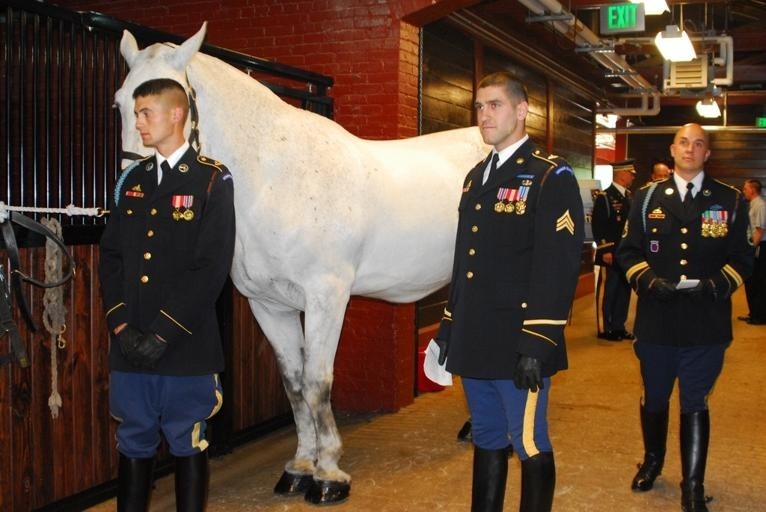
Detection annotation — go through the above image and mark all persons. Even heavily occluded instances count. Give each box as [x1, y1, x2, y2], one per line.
[96, 75, 234, 511]
[591, 156, 765, 342]
[612, 120, 759, 512]
[434, 64, 585, 511]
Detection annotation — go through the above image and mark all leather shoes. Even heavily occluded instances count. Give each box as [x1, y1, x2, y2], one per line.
[618, 329, 634, 340]
[746, 319, 765, 324]
[737, 315, 749, 320]
[598, 330, 622, 341]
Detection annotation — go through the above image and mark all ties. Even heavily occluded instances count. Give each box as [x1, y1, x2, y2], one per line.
[625, 189, 630, 200]
[159, 160, 172, 185]
[684, 182, 695, 206]
[488, 152, 499, 179]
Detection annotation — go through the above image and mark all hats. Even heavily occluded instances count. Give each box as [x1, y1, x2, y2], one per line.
[608, 159, 638, 175]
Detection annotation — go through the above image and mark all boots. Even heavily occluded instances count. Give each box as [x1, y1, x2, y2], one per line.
[116, 452, 153, 512]
[469, 446, 508, 512]
[520, 452, 555, 512]
[679, 409, 711, 512]
[631, 396, 669, 492]
[172, 448, 208, 512]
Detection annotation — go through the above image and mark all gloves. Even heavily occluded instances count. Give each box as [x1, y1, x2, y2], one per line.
[513, 356, 544, 393]
[433, 338, 449, 366]
[685, 276, 717, 306]
[115, 324, 158, 372]
[123, 331, 169, 368]
[649, 279, 680, 302]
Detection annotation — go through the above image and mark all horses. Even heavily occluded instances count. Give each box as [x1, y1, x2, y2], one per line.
[111, 21, 493, 506]
